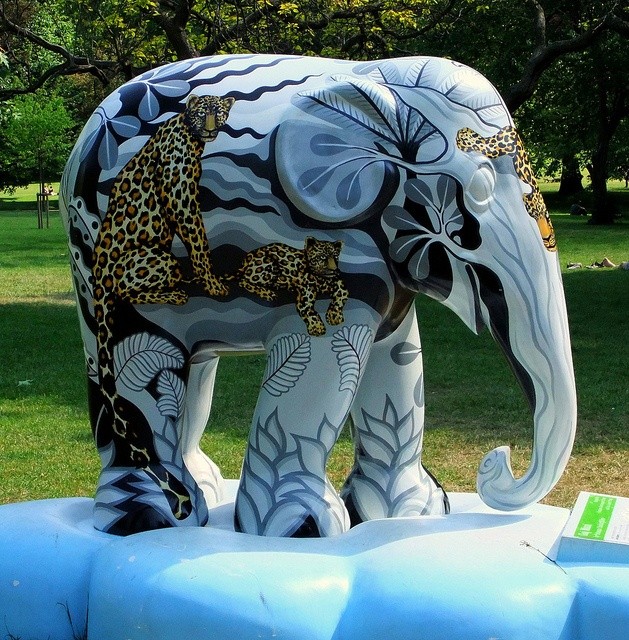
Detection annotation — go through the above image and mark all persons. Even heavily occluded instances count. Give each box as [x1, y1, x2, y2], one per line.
[601, 257, 629, 270]
